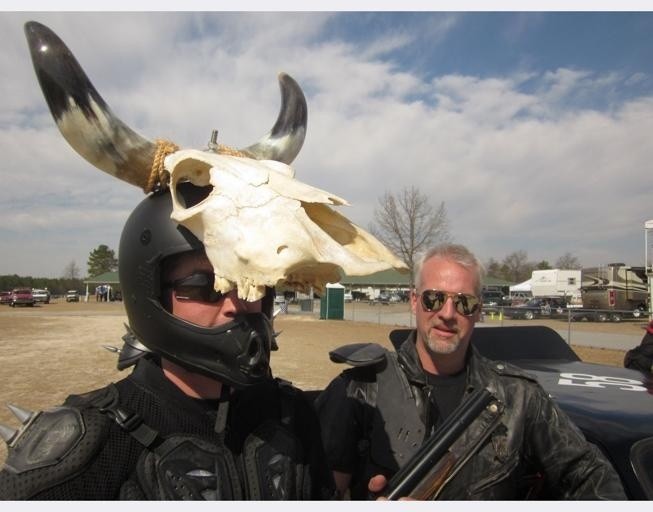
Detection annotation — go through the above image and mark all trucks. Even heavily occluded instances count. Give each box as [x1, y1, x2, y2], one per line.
[483, 263, 652, 323]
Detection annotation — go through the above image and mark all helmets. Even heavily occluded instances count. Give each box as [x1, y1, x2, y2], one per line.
[103, 185, 279, 390]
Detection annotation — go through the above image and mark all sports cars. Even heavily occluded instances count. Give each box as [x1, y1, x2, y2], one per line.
[358, 323, 652, 501]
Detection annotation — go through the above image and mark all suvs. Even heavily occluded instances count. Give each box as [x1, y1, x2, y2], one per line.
[344, 290, 352, 303]
[65, 289, 79, 302]
[11, 287, 32, 307]
[31, 290, 50, 304]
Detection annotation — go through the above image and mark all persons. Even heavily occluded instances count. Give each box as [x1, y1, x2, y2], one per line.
[622, 317, 653, 378]
[94, 283, 116, 302]
[1, 19, 414, 501]
[315, 242, 627, 501]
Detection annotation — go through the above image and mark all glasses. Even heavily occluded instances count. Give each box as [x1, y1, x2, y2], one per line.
[415, 290, 483, 319]
[156, 269, 221, 307]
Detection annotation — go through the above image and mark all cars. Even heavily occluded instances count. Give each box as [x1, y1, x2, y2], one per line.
[375, 292, 408, 305]
[0, 292, 12, 304]
